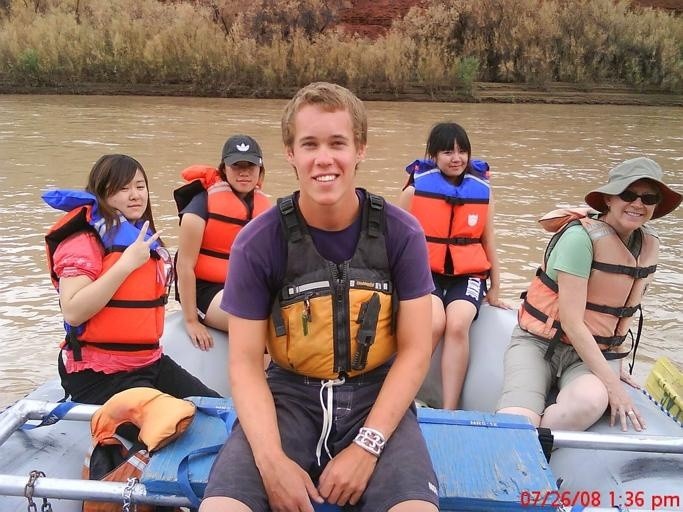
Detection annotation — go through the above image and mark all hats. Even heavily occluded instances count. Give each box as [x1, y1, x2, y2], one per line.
[222, 135, 263, 166]
[584, 157, 683, 220]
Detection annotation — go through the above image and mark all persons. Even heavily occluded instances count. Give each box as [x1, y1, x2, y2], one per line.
[45, 153, 224, 405]
[495, 156, 683, 431]
[394, 120, 514, 410]
[172, 133, 274, 352]
[193, 80, 441, 512]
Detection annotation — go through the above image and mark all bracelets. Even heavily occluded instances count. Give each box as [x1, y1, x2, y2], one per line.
[351, 426, 387, 458]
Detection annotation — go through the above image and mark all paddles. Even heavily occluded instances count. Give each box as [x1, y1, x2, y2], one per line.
[644, 357, 682, 425]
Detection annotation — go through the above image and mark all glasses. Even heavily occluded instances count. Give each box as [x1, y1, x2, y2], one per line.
[616, 190, 661, 205]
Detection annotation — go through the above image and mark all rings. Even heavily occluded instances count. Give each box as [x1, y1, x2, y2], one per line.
[627, 411, 635, 416]
